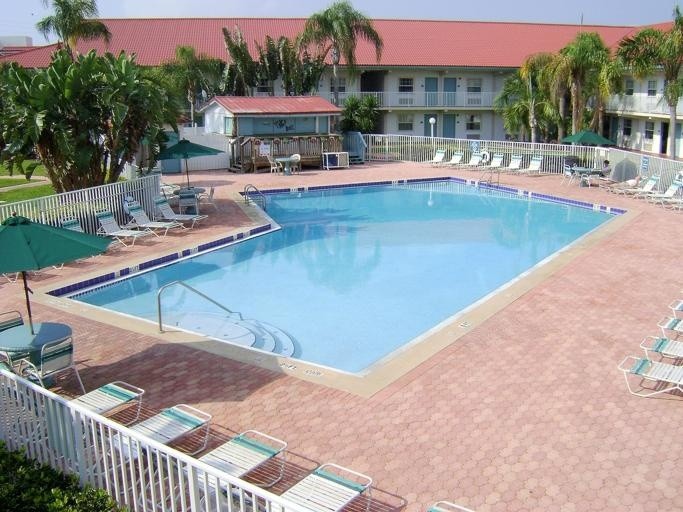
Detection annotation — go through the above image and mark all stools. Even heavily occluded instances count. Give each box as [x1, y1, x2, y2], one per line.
[619, 302, 683, 401]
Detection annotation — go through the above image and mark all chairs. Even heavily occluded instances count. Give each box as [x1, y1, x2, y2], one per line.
[61, 186, 215, 248]
[424, 149, 544, 179]
[268, 153, 301, 175]
[564, 162, 681, 211]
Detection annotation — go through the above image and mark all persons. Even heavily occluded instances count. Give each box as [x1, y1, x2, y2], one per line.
[608, 175, 641, 189]
[597, 160, 611, 177]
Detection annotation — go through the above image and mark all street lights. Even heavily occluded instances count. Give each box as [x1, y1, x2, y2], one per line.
[429, 117, 436, 137]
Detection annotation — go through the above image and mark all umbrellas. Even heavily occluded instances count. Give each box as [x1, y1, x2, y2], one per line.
[145, 137, 226, 190]
[0, 211, 112, 334]
[561, 129, 616, 168]
